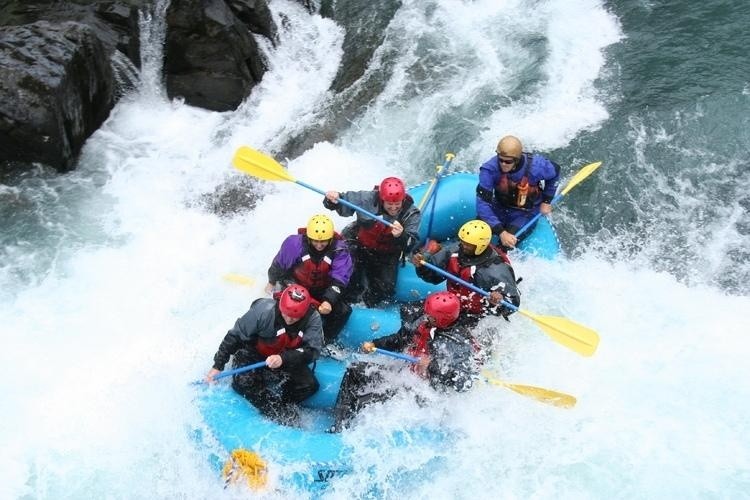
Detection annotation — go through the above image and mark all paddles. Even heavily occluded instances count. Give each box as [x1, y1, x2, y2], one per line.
[371, 347, 577, 410]
[514, 162, 603, 238]
[420, 260, 599, 356]
[234, 147, 396, 227]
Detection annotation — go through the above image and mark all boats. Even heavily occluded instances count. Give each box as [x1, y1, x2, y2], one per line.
[182, 170, 564, 500]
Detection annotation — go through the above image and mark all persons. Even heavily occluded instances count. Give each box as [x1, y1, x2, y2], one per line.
[320, 175, 421, 309]
[332, 289, 479, 433]
[205, 285, 326, 430]
[402, 218, 521, 329]
[266, 211, 356, 365]
[472, 135, 560, 255]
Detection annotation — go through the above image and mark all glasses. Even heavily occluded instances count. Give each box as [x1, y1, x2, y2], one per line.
[498, 158, 514, 164]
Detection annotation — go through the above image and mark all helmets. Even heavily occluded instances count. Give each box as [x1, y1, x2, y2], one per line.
[380, 177, 405, 201]
[424, 292, 460, 327]
[279, 284, 311, 318]
[307, 214, 334, 241]
[458, 219, 492, 256]
[495, 136, 522, 159]
